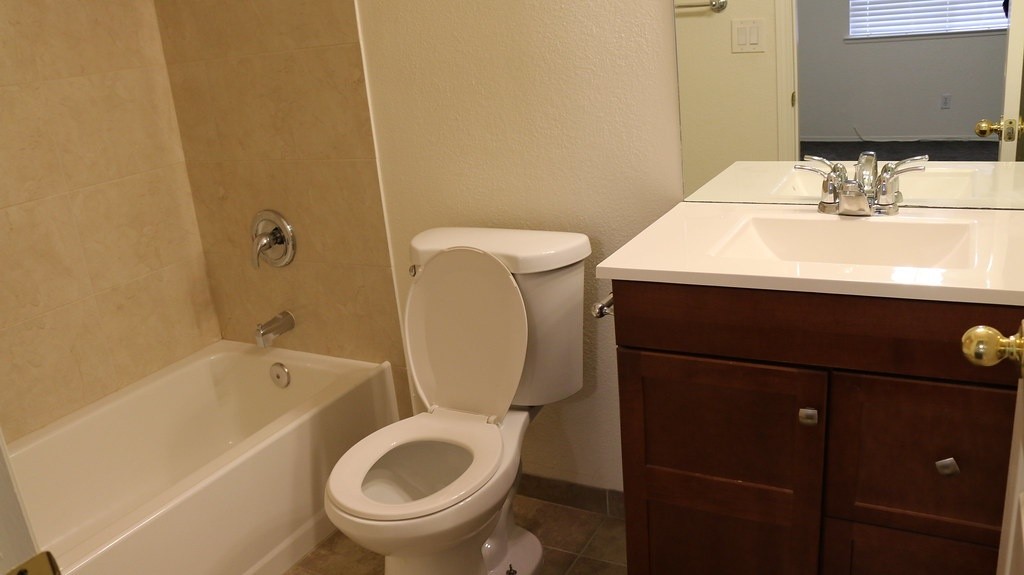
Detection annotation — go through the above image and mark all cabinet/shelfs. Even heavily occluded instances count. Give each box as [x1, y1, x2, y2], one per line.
[612, 279, 1023, 575]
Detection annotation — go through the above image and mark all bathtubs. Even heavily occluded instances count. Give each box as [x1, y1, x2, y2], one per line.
[4, 338, 401, 575]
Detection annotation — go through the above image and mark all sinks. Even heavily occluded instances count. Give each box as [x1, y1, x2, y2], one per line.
[707, 212, 982, 278]
[765, 162, 1001, 208]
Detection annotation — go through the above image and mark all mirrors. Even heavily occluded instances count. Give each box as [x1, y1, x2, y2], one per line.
[673, 1, 1024, 210]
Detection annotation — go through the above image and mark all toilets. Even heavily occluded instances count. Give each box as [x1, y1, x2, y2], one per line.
[322, 225, 592, 575]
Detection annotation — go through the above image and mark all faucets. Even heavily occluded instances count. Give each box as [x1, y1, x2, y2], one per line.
[255, 309, 294, 349]
[793, 164, 927, 216]
[801, 151, 929, 202]
